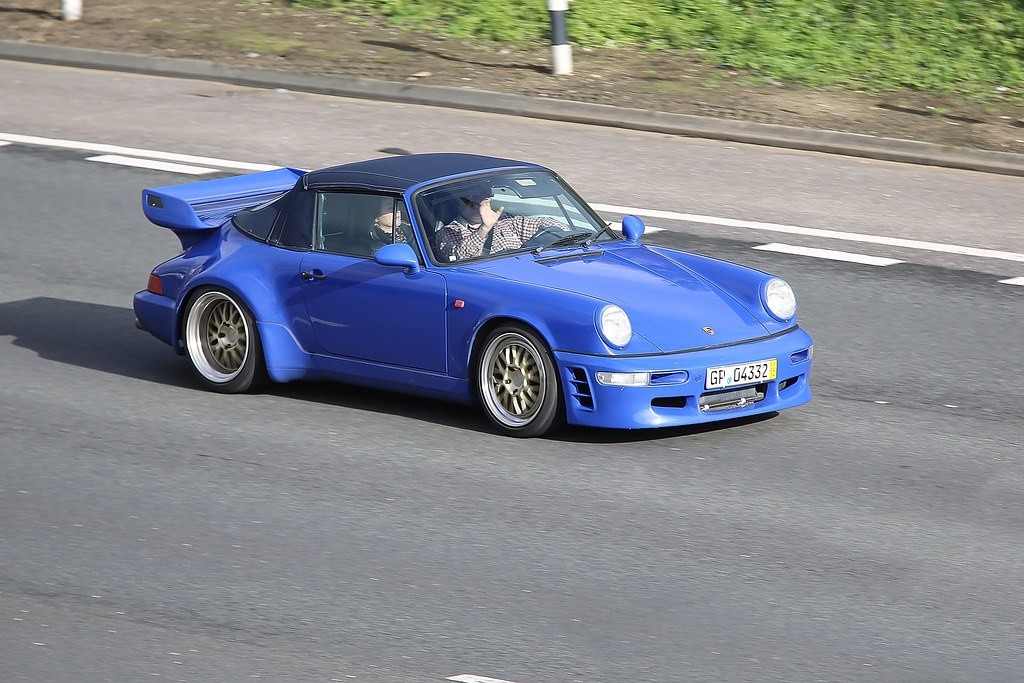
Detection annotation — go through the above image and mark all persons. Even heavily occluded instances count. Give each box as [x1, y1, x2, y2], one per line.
[356, 196, 425, 266]
[430, 177, 572, 263]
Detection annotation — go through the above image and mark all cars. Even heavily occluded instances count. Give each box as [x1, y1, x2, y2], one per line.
[132, 153, 815, 440]
